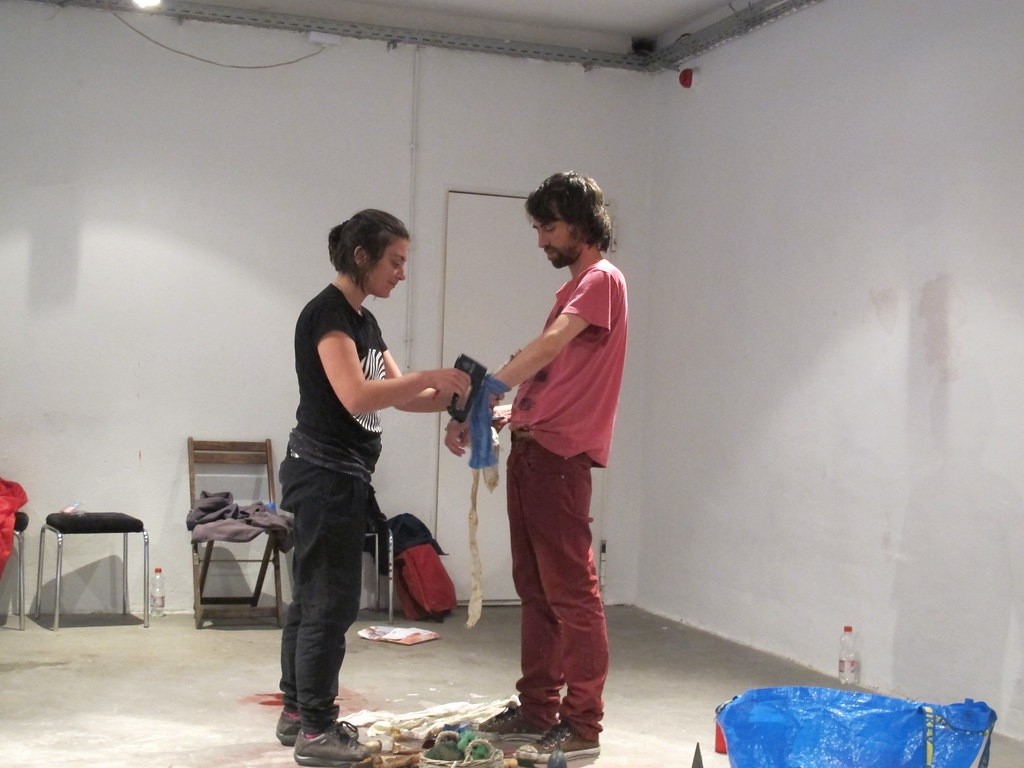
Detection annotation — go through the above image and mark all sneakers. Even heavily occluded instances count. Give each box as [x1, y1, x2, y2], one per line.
[516, 722, 600, 763]
[294, 721, 382, 767]
[276, 707, 303, 746]
[465, 701, 560, 741]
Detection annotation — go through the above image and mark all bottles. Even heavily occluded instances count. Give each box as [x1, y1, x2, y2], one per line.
[150, 567, 167, 618]
[838, 625, 859, 687]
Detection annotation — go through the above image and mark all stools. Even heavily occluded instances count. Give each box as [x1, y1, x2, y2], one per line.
[14, 511, 29, 631]
[35, 513, 149, 631]
[366, 527, 394, 626]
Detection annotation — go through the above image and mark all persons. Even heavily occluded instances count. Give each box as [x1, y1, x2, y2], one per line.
[445, 170, 630, 761]
[275, 208, 504, 768]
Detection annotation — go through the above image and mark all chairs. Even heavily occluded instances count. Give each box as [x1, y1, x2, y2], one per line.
[187, 438, 284, 630]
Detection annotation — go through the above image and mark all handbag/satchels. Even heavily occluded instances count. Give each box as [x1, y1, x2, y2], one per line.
[393, 542, 457, 623]
